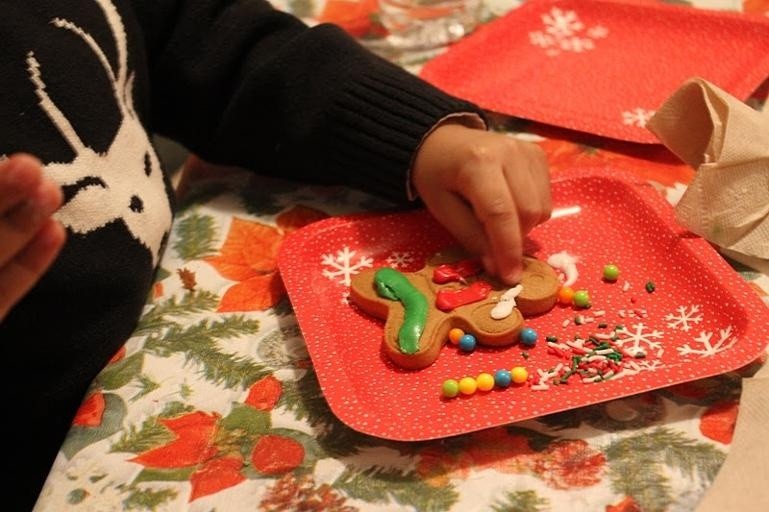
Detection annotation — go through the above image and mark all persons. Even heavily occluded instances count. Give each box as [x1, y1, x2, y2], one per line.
[1, 1, 555, 510]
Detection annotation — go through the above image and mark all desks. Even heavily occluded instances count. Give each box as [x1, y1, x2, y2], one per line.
[30, 0, 769, 512]
